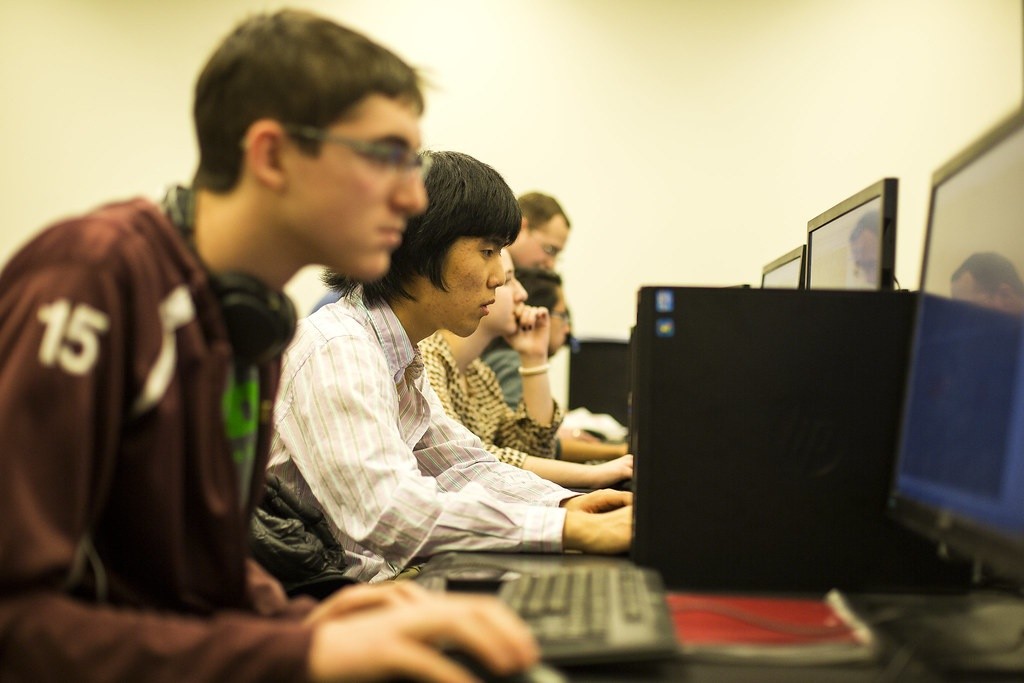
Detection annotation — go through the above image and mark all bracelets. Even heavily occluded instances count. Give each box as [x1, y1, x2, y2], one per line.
[517, 366, 548, 376]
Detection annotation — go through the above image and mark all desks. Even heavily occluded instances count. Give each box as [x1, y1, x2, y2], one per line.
[395, 483, 1024, 683]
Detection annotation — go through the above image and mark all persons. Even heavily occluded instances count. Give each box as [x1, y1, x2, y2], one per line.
[1, 8, 544, 683]
[272, 151, 632, 591]
[308, 192, 570, 314]
[851, 211, 881, 284]
[419, 245, 632, 487]
[950, 251, 1024, 318]
[482, 268, 630, 460]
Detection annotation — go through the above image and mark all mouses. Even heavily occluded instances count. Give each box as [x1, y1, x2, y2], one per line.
[383, 643, 570, 683]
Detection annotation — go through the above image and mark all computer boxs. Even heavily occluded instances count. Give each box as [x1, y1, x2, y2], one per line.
[629, 287, 981, 599]
[567, 335, 630, 436]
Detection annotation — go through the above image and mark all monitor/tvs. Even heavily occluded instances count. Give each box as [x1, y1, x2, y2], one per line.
[763, 103, 1024, 683]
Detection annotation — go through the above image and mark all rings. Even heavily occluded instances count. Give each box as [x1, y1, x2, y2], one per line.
[572, 429, 580, 437]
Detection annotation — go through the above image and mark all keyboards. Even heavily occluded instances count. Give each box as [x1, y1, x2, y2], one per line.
[499, 568, 684, 683]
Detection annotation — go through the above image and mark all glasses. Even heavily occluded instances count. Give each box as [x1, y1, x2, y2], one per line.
[551, 305, 570, 326]
[290, 130, 432, 187]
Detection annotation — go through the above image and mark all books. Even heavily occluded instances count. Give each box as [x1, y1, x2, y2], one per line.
[668, 594, 872, 666]
